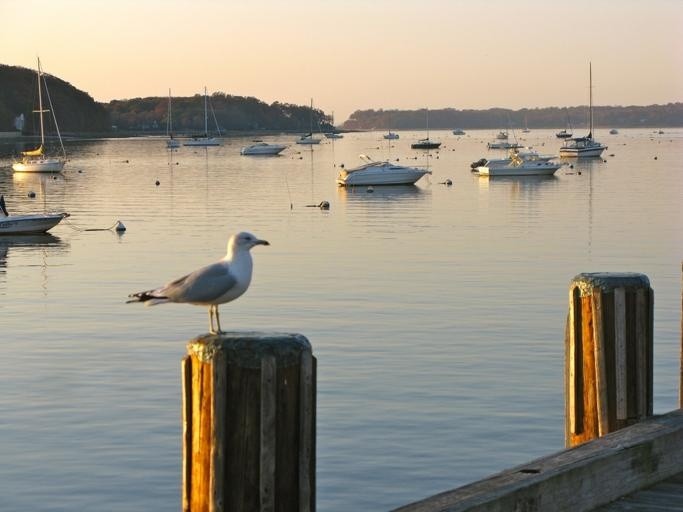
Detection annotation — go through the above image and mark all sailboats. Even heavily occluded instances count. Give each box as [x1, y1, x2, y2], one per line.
[383, 105, 440, 149]
[167, 87, 224, 148]
[10, 58, 66, 172]
[296, 98, 342, 144]
[561, 62, 607, 157]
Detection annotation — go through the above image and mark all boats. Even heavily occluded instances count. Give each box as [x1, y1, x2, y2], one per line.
[0, 195, 69, 236]
[487, 127, 529, 148]
[240, 144, 285, 154]
[555, 131, 572, 137]
[479, 154, 559, 177]
[338, 154, 431, 186]
[452, 127, 464, 135]
[609, 129, 617, 134]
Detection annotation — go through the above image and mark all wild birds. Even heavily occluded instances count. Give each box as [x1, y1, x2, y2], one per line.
[125, 230, 271, 336]
